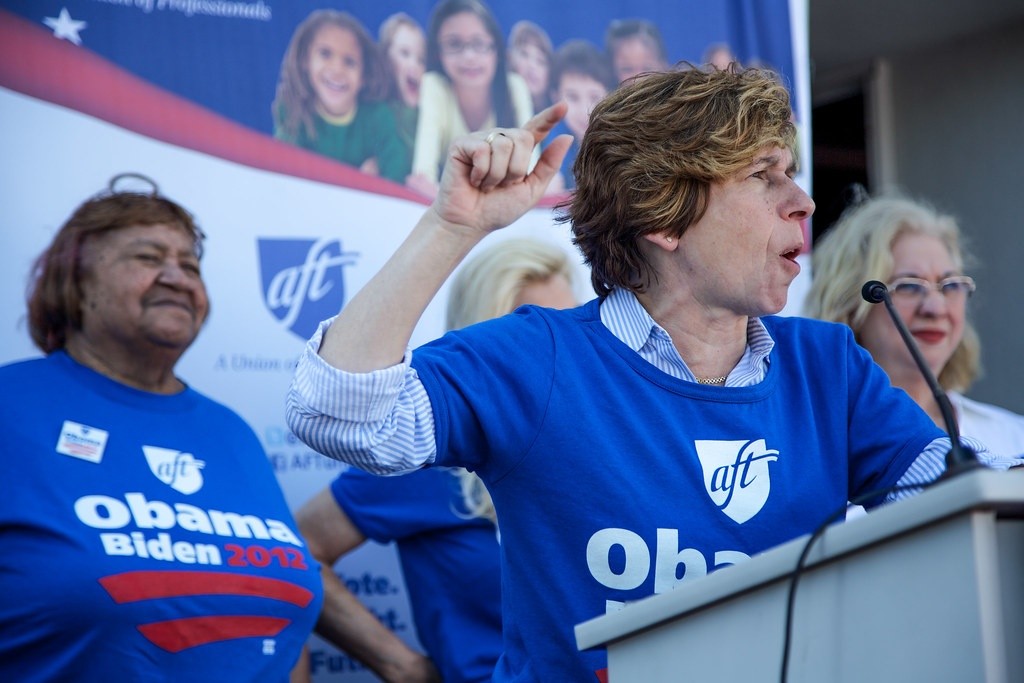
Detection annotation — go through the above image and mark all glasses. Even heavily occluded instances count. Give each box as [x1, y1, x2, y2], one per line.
[886, 275, 975, 306]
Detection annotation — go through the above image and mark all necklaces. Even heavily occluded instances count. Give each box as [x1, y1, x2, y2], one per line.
[695, 372, 727, 387]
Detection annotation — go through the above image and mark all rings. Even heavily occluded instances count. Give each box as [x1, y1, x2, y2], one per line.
[484, 131, 505, 145]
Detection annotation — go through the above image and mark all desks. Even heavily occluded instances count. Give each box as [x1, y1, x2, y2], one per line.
[574, 472, 1023, 682]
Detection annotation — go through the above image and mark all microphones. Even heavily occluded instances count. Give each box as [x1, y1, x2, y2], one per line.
[862, 281, 994, 489]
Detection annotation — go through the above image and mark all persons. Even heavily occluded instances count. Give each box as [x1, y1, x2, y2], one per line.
[268, 7, 409, 185]
[293, 236, 579, 683]
[405, 1, 545, 203]
[504, 18, 554, 120]
[283, 56, 1022, 683]
[701, 42, 736, 72]
[2, 193, 325, 681]
[538, 41, 617, 192]
[799, 196, 1024, 518]
[606, 17, 668, 85]
[373, 11, 430, 152]
